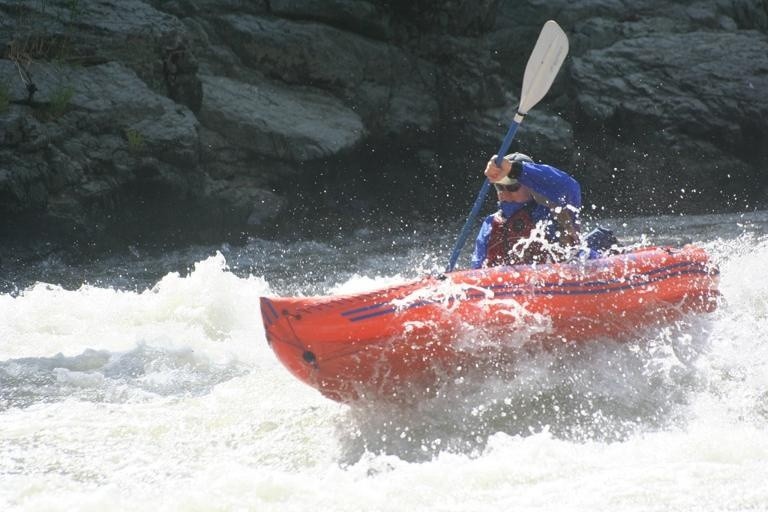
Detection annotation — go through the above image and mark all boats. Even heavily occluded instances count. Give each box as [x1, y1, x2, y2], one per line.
[259, 242, 720, 411]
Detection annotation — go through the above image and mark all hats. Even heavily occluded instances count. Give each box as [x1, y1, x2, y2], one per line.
[489, 152, 535, 184]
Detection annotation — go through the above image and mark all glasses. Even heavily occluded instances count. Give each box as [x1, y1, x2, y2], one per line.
[494, 183, 521, 191]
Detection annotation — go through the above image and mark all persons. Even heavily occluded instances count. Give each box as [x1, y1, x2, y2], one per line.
[472, 151, 617, 269]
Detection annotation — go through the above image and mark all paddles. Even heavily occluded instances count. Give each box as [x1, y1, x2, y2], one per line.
[444, 21, 569, 272]
